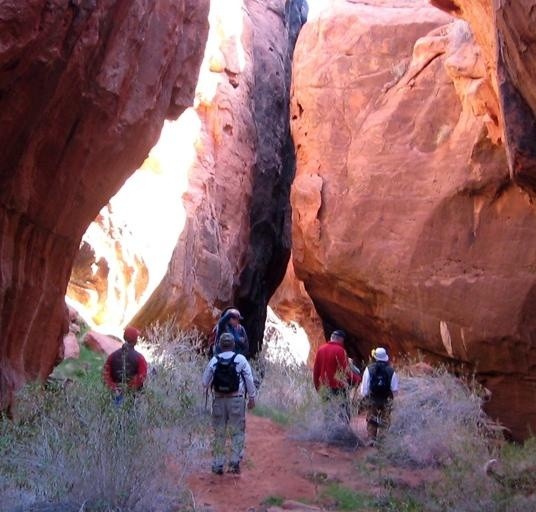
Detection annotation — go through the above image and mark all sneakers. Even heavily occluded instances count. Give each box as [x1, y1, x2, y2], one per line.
[227, 463, 240, 471]
[212, 465, 223, 474]
[366, 436, 383, 446]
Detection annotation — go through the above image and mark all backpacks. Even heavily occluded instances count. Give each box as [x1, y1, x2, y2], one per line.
[370, 364, 390, 395]
[213, 354, 239, 393]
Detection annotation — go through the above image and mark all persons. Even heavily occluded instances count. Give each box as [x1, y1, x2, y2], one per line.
[355, 348, 400, 448]
[210, 309, 250, 408]
[103, 328, 148, 446]
[312, 330, 362, 447]
[201, 332, 256, 475]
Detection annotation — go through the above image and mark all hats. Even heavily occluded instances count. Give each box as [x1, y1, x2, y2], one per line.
[333, 330, 347, 339]
[371, 347, 389, 362]
[126, 328, 142, 338]
[221, 306, 237, 317]
[220, 333, 235, 345]
